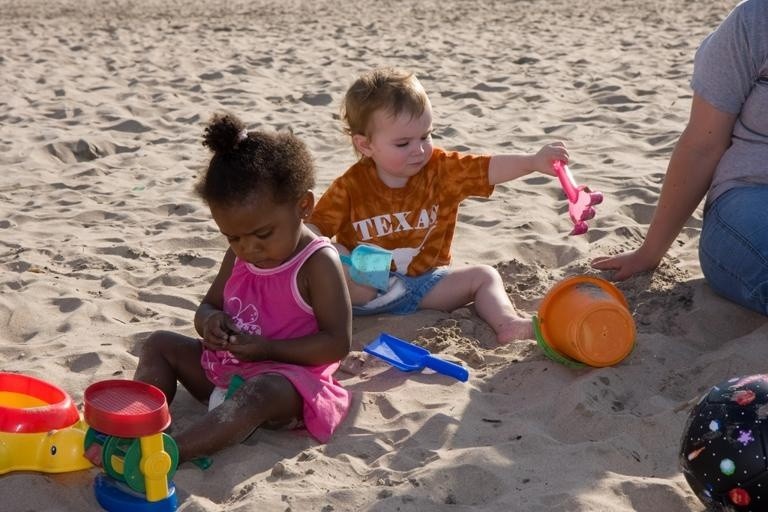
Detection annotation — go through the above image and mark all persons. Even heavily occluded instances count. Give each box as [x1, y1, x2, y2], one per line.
[591, 0, 767, 315]
[304, 68, 570, 345]
[132, 113, 353, 470]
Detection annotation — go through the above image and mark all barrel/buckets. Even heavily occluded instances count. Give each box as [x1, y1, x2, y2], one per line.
[532, 275, 637, 369]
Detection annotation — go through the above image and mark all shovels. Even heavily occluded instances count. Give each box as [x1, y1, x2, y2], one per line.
[363, 332, 468, 382]
[340, 245, 393, 292]
